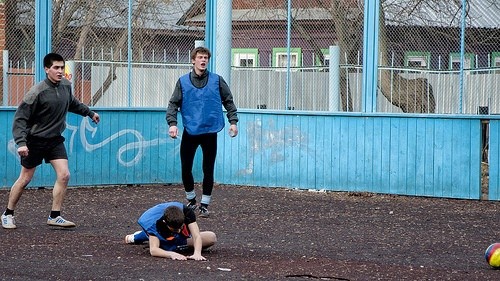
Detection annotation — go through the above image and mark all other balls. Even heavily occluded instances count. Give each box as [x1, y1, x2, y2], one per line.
[485, 242, 500, 267]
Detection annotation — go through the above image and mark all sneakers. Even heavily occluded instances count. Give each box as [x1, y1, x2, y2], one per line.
[47, 215, 76, 228]
[187, 202, 197, 210]
[199, 206, 209, 218]
[125, 231, 142, 244]
[1, 210, 16, 228]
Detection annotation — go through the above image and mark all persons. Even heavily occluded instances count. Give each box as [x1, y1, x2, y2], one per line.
[1, 53, 100, 228]
[125, 202, 216, 260]
[165, 47, 239, 218]
